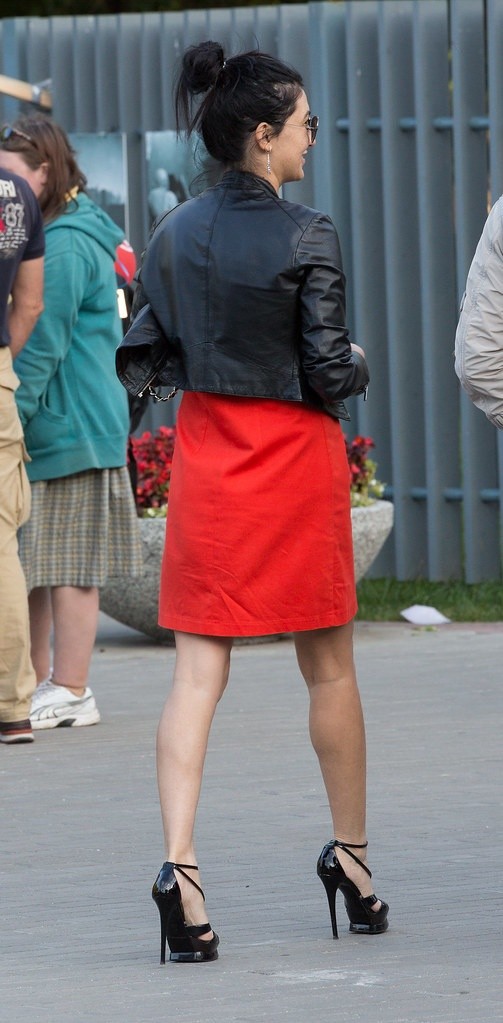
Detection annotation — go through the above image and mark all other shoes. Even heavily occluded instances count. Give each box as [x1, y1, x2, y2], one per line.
[0, 719, 34, 743]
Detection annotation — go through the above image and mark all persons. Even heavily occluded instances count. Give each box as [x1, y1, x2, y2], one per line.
[0, 113, 145, 728]
[115, 43, 388, 964]
[453, 196, 503, 431]
[0, 168, 46, 744]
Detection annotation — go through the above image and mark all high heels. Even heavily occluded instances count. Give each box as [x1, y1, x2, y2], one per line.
[317, 838, 389, 940]
[151, 862, 219, 965]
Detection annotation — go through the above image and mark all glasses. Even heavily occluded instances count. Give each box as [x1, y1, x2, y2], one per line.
[0, 127, 38, 149]
[249, 116, 319, 144]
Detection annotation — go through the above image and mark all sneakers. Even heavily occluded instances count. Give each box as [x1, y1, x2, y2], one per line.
[29, 676, 100, 730]
